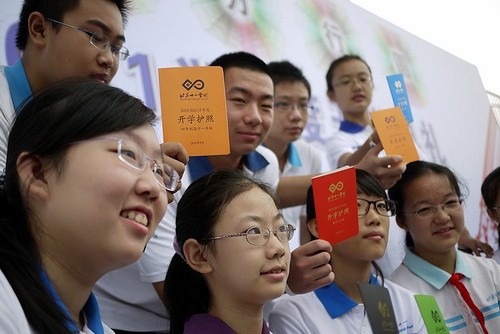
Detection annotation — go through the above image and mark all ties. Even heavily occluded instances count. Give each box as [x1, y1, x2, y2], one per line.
[449, 273, 489, 334]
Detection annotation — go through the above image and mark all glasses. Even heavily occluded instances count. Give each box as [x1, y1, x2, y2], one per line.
[203, 225, 297, 247]
[404, 198, 466, 220]
[356, 197, 398, 218]
[43, 17, 130, 60]
[71, 136, 181, 191]
[273, 99, 314, 114]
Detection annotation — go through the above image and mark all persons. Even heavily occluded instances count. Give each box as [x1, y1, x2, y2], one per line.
[264, 57, 333, 254]
[0, 75, 170, 334]
[325, 53, 495, 278]
[91, 49, 335, 334]
[0, 0, 192, 202]
[481, 165, 500, 264]
[163, 171, 292, 334]
[383, 159, 500, 334]
[268, 166, 429, 333]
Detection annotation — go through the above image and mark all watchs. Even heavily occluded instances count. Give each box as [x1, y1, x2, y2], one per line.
[368, 135, 376, 148]
